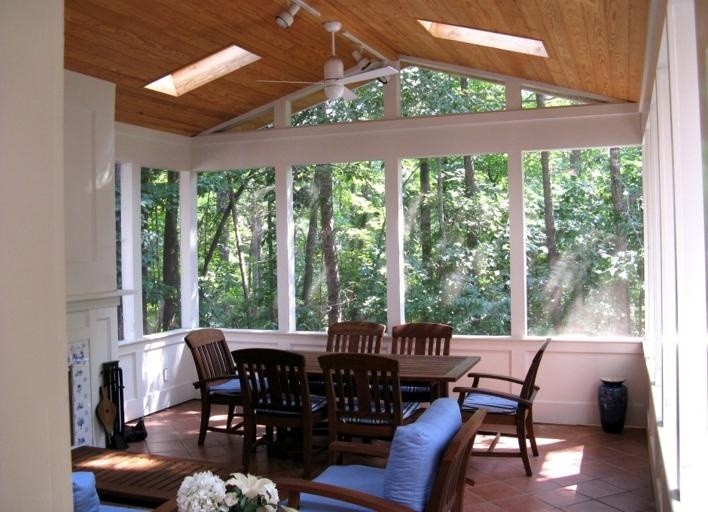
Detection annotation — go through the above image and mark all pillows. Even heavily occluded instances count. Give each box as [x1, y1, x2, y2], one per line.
[384, 398, 464, 512]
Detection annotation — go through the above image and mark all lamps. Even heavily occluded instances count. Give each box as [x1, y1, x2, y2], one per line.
[274, 2, 301, 30]
[350, 48, 375, 73]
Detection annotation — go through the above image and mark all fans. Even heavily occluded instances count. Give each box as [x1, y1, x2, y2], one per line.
[255, 20, 399, 103]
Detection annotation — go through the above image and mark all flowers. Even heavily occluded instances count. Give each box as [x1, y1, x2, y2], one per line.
[177, 470, 280, 512]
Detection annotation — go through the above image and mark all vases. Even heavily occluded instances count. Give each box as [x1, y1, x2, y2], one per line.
[596, 377, 630, 435]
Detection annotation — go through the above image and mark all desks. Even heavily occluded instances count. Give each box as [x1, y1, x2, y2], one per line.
[71, 446, 229, 507]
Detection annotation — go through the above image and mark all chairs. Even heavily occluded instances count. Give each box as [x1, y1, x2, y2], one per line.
[70, 472, 178, 512]
[387, 322, 453, 402]
[231, 348, 329, 478]
[450, 338, 550, 477]
[275, 398, 487, 512]
[184, 328, 270, 447]
[322, 320, 386, 396]
[317, 352, 424, 468]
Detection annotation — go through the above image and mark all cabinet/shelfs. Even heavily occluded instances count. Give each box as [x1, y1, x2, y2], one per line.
[290, 350, 482, 401]
